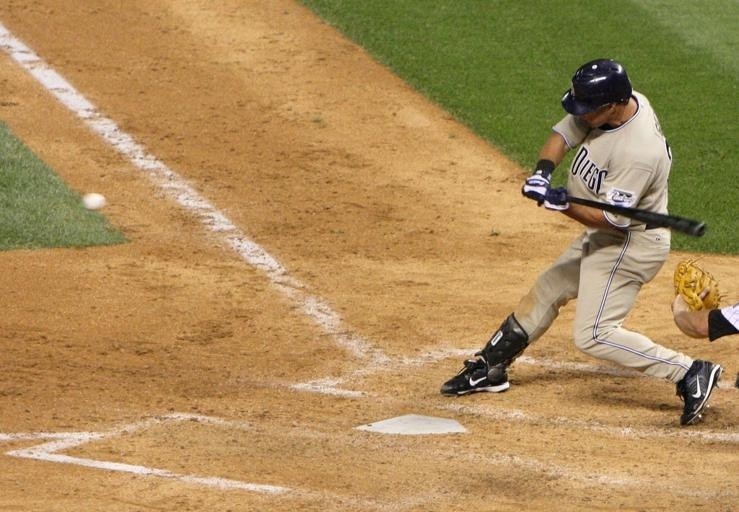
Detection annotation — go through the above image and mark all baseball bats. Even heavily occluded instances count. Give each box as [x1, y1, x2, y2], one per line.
[545, 188, 707, 239]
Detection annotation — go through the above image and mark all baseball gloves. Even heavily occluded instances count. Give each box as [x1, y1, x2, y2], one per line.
[672, 261, 722, 316]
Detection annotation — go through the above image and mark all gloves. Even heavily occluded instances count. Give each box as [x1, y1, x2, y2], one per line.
[523, 160, 570, 210]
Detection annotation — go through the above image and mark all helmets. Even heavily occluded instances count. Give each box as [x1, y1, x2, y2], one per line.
[561, 58, 632, 114]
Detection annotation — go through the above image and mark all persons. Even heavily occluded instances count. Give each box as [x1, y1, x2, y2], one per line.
[670, 257, 739, 342]
[440, 58, 725, 426]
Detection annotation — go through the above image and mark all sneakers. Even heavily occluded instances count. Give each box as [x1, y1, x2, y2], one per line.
[440, 352, 509, 396]
[676, 359, 724, 426]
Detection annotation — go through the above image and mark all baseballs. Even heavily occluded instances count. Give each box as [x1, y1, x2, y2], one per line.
[81, 193, 107, 210]
